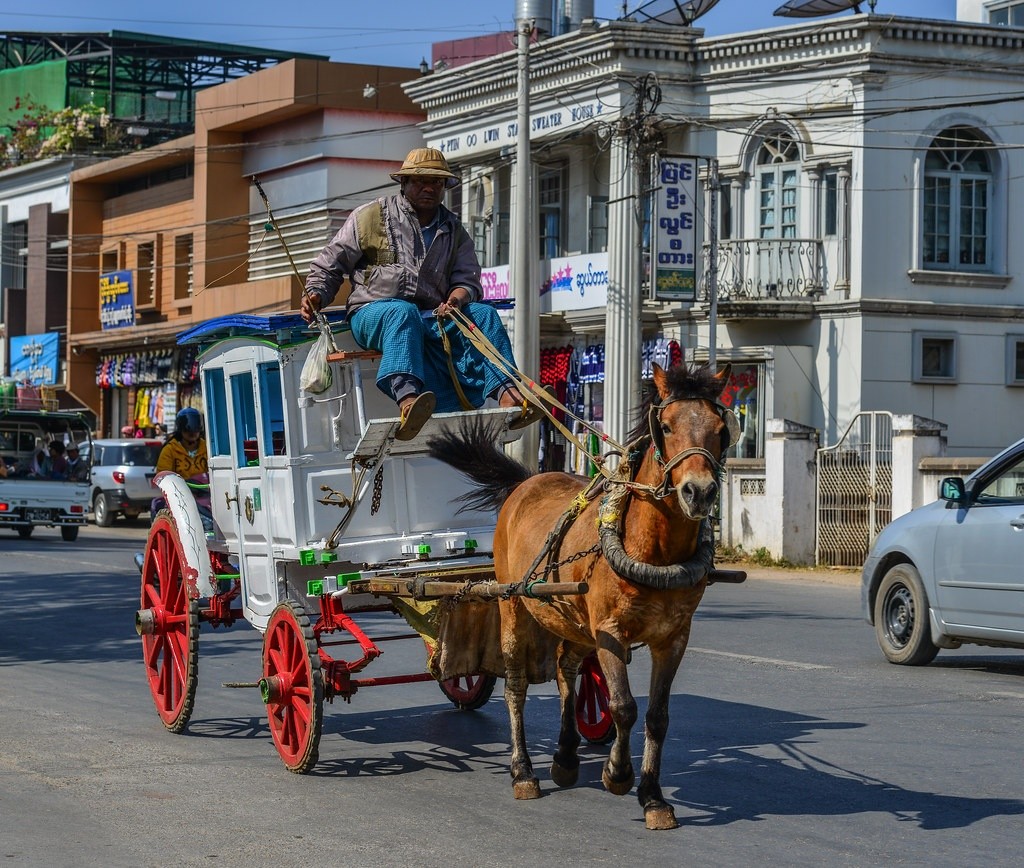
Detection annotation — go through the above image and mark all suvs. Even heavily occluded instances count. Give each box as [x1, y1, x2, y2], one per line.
[59, 437, 168, 528]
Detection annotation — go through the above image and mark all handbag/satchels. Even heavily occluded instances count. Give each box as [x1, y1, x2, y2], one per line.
[300, 327, 333, 394]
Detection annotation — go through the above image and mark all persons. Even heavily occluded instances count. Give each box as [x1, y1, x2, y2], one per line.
[155, 380, 214, 529]
[300, 148, 557, 441]
[44, 441, 88, 482]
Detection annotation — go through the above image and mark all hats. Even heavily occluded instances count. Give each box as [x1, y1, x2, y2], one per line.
[48, 441, 65, 453]
[65, 443, 79, 451]
[121, 426, 133, 434]
[389, 148, 463, 192]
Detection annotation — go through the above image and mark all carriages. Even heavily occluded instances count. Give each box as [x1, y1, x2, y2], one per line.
[133, 301, 748, 832]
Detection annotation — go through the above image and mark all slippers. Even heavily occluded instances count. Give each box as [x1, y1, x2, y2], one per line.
[512, 386, 557, 430]
[395, 392, 435, 441]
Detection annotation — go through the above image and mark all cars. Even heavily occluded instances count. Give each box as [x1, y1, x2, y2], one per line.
[860, 437, 1024, 667]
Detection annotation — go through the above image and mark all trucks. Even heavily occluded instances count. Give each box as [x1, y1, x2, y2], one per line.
[0, 407, 96, 542]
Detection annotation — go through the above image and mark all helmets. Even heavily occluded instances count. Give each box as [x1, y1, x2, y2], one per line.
[176, 407, 203, 432]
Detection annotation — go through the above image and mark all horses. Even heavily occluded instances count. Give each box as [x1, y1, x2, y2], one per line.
[424, 361, 732, 830]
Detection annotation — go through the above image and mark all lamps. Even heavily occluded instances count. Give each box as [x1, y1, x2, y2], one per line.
[363, 81, 403, 101]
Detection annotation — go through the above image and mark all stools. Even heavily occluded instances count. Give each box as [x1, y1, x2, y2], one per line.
[327, 349, 386, 445]
